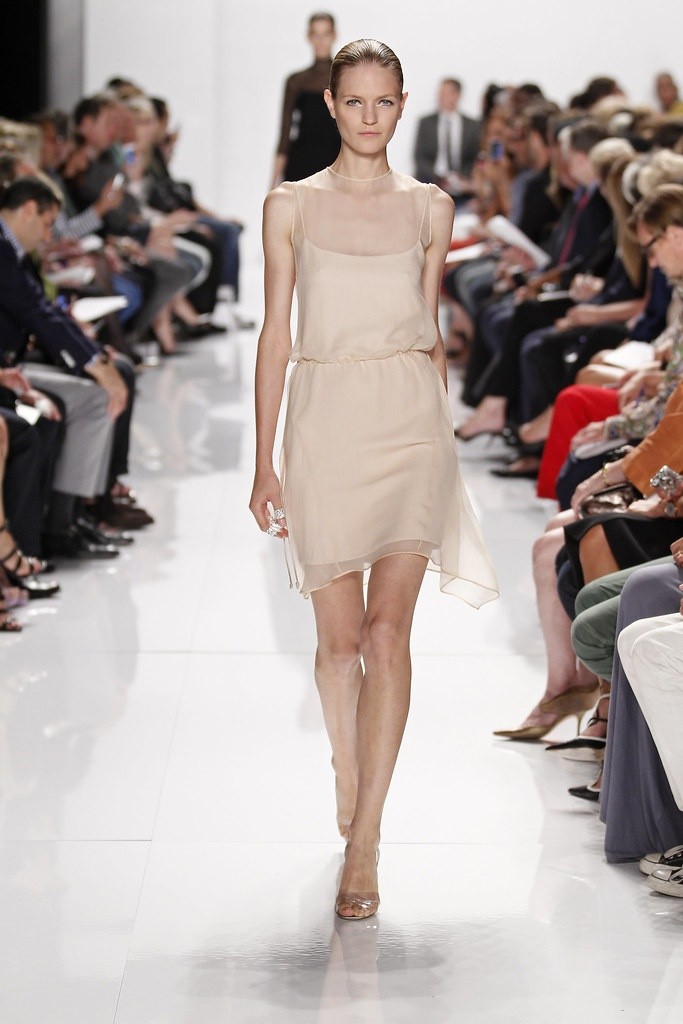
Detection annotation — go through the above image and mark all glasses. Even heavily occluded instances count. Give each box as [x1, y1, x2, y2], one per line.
[640, 234, 660, 257]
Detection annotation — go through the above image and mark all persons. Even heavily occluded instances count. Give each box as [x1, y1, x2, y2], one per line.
[249, 38, 500, 920]
[0, 77, 254, 631]
[409, 72, 683, 898]
[271, 13, 341, 189]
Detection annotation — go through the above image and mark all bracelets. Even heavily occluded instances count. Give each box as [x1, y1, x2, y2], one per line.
[601, 463, 611, 488]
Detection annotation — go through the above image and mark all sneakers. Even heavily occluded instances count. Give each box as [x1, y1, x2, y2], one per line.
[646, 866, 683, 898]
[639, 837, 683, 876]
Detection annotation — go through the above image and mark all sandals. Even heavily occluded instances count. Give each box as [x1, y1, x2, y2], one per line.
[336, 814, 355, 843]
[489, 453, 539, 479]
[0, 521, 54, 576]
[334, 841, 380, 922]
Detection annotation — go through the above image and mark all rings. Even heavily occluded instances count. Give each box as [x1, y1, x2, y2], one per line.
[265, 508, 285, 537]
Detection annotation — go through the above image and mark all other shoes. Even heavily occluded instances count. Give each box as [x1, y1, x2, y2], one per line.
[0, 590, 21, 631]
[76, 518, 133, 546]
[43, 525, 123, 560]
[105, 503, 154, 530]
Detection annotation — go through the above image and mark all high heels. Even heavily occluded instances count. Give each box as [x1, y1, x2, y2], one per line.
[567, 760, 601, 800]
[504, 405, 546, 453]
[453, 396, 506, 447]
[545, 694, 609, 752]
[493, 680, 596, 739]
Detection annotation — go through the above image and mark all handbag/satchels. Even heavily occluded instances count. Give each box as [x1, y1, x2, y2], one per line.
[579, 483, 639, 520]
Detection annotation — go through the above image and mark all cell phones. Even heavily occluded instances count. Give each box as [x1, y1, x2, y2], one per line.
[487, 139, 502, 164]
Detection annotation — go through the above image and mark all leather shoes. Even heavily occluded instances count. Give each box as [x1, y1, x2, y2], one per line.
[12, 577, 59, 598]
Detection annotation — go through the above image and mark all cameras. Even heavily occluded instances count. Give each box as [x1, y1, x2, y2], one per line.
[649, 465, 683, 495]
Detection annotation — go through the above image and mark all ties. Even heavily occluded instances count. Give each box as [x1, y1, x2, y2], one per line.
[443, 120, 454, 170]
